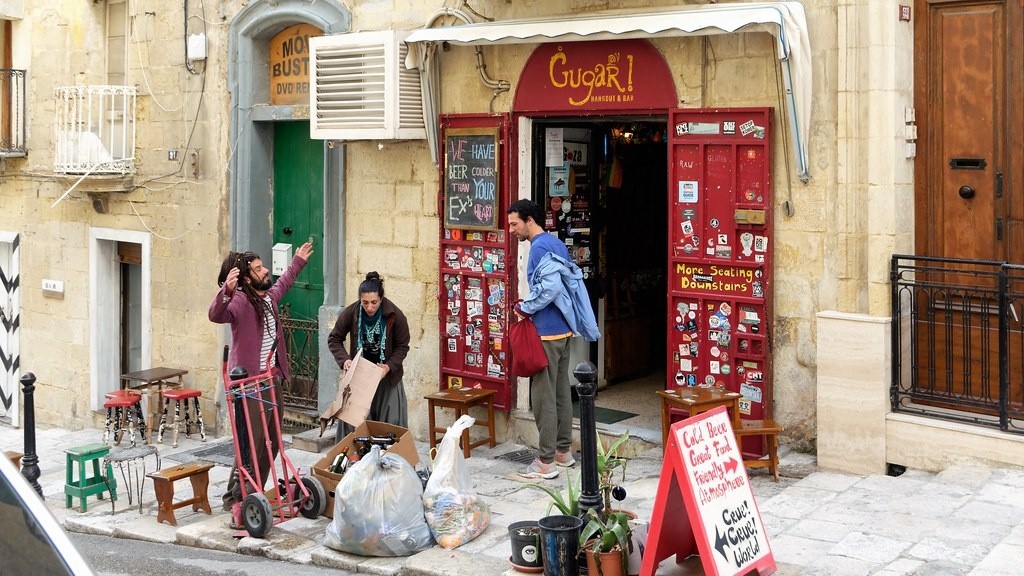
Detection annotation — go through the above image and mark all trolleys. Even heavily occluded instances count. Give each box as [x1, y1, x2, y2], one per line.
[222, 335, 327, 539]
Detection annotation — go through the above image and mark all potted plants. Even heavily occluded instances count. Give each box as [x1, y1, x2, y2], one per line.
[501, 427, 638, 576]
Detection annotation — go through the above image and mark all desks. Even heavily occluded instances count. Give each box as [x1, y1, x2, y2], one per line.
[102, 445, 161, 516]
[116, 367, 188, 445]
[423, 387, 498, 460]
[656, 385, 744, 467]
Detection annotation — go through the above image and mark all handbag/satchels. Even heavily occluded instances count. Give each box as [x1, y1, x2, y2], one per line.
[422, 414, 491, 551]
[509, 298, 548, 378]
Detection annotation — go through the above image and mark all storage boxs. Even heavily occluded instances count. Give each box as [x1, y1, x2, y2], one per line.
[311, 420, 419, 519]
[263, 484, 298, 517]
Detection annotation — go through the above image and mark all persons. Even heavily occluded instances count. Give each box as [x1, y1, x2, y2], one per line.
[327, 270, 416, 473]
[207, 241, 314, 515]
[505, 197, 602, 480]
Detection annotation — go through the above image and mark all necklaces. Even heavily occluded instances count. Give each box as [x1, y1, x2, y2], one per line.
[357, 303, 387, 364]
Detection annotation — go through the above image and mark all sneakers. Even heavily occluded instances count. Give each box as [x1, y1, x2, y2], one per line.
[555, 450, 576, 467]
[516, 456, 560, 479]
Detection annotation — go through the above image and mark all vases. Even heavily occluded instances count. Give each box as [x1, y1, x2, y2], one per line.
[508, 521, 543, 567]
[537, 515, 583, 576]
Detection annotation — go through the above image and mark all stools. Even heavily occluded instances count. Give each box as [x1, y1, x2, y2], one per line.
[3, 451, 25, 471]
[63, 443, 118, 513]
[728, 420, 784, 483]
[102, 389, 206, 449]
[146, 461, 215, 527]
